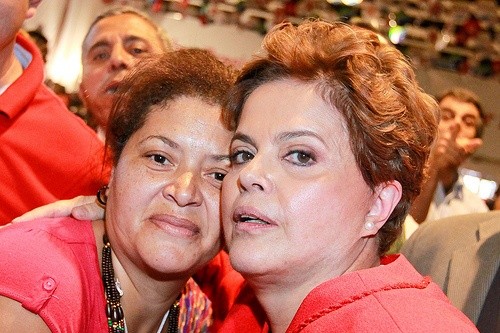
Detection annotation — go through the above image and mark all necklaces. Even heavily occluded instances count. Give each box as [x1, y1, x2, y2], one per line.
[102, 232, 182, 333]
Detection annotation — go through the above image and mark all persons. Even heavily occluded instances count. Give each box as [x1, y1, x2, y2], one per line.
[405, 88, 500, 333]
[0, 16, 480, 333]
[0, 0, 168, 223]
[0, 46, 246, 333]
